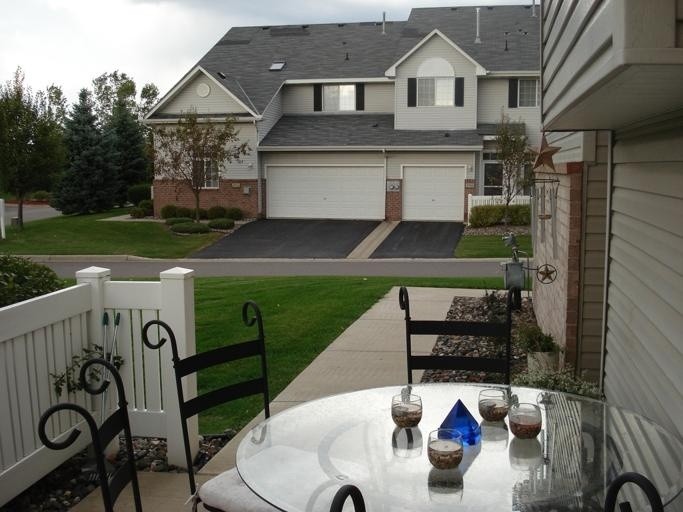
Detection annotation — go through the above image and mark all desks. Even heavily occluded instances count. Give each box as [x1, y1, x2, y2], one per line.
[235, 381, 683, 512]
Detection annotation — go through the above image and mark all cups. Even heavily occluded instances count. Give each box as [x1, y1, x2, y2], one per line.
[508, 402, 542, 440]
[427, 428, 463, 469]
[391, 393, 423, 428]
[477, 388, 509, 421]
